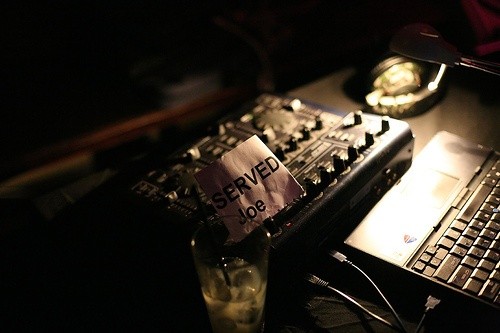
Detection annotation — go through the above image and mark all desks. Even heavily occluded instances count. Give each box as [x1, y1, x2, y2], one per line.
[270, 70, 498, 333]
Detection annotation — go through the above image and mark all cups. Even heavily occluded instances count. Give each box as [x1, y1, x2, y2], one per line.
[191, 217, 269, 333]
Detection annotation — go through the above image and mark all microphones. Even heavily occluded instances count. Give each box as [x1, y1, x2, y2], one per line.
[390, 21, 500, 76]
